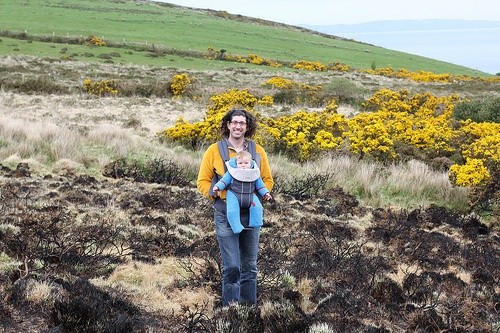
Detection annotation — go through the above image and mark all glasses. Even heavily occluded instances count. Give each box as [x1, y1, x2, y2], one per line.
[230, 121, 247, 126]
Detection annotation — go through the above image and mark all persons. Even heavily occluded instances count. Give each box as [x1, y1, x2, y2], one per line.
[196, 110, 274, 308]
[213, 151, 272, 234]
